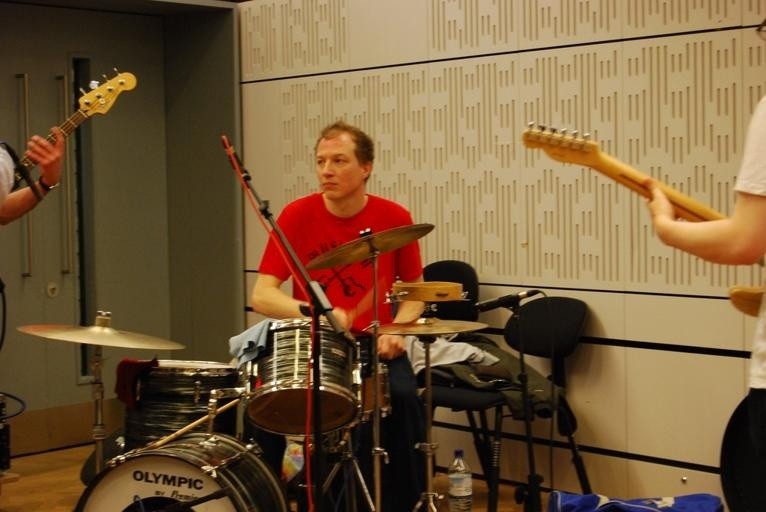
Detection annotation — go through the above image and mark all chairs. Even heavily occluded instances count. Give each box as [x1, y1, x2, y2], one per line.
[417, 260, 497, 489]
[425, 297, 593, 511]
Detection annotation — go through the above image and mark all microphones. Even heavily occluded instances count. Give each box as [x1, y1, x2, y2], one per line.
[475, 291, 540, 313]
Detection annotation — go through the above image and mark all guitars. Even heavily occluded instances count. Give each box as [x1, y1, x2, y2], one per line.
[522, 123, 766, 317]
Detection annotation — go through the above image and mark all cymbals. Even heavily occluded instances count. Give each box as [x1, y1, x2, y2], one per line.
[364, 318, 488, 334]
[305, 222, 435, 269]
[17, 324, 186, 349]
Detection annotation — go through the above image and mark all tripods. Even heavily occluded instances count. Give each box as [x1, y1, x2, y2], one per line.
[413, 343, 445, 511]
[321, 435, 377, 512]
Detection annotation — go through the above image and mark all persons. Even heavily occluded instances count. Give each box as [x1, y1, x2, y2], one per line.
[250, 122, 438, 511]
[0, 125, 68, 229]
[645, 17, 765, 510]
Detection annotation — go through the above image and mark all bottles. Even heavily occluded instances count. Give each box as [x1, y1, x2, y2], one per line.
[446, 448, 474, 512]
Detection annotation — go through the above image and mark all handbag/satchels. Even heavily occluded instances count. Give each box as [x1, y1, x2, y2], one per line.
[548, 490, 724, 512]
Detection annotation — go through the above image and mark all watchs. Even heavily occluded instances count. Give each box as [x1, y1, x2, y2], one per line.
[39, 176, 60, 192]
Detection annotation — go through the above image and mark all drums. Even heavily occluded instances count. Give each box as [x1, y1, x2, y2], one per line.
[126, 360, 247, 454]
[346, 362, 392, 428]
[74, 431, 288, 512]
[245, 318, 361, 437]
[384, 281, 468, 304]
